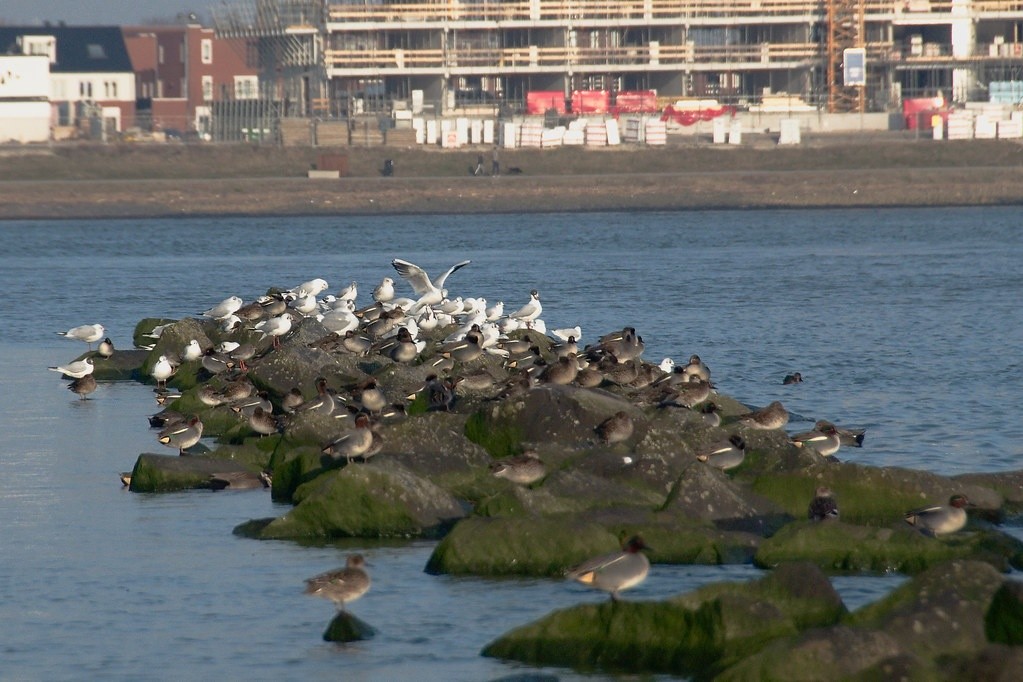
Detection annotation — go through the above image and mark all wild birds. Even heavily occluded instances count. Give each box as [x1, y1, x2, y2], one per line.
[813, 420, 867, 448]
[98, 337, 115, 361]
[57, 324, 109, 350]
[783, 372, 803, 386]
[736, 401, 790, 443]
[116, 277, 753, 492]
[807, 487, 840, 526]
[67, 374, 97, 400]
[47, 355, 97, 380]
[786, 424, 840, 458]
[561, 534, 655, 604]
[301, 552, 376, 614]
[902, 493, 979, 541]
[391, 258, 472, 322]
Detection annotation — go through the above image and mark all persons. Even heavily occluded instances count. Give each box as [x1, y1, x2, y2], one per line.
[380, 146, 510, 182]
